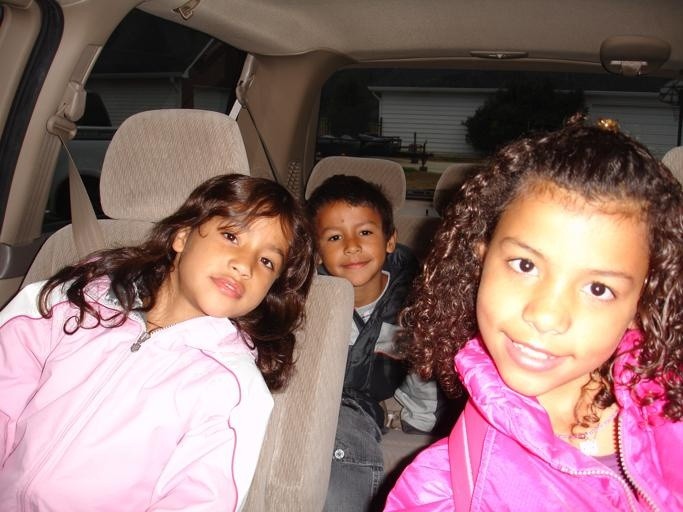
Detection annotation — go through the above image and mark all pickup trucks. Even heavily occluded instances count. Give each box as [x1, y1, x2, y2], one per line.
[47, 91, 117, 220]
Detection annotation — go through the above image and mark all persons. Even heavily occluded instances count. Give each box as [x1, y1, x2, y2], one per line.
[301, 172, 439, 511]
[0, 171, 323, 512]
[378, 109, 682, 512]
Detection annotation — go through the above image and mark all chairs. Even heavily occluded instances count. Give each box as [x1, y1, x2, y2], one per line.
[19, 110, 355, 512]
[304, 145, 682, 279]
